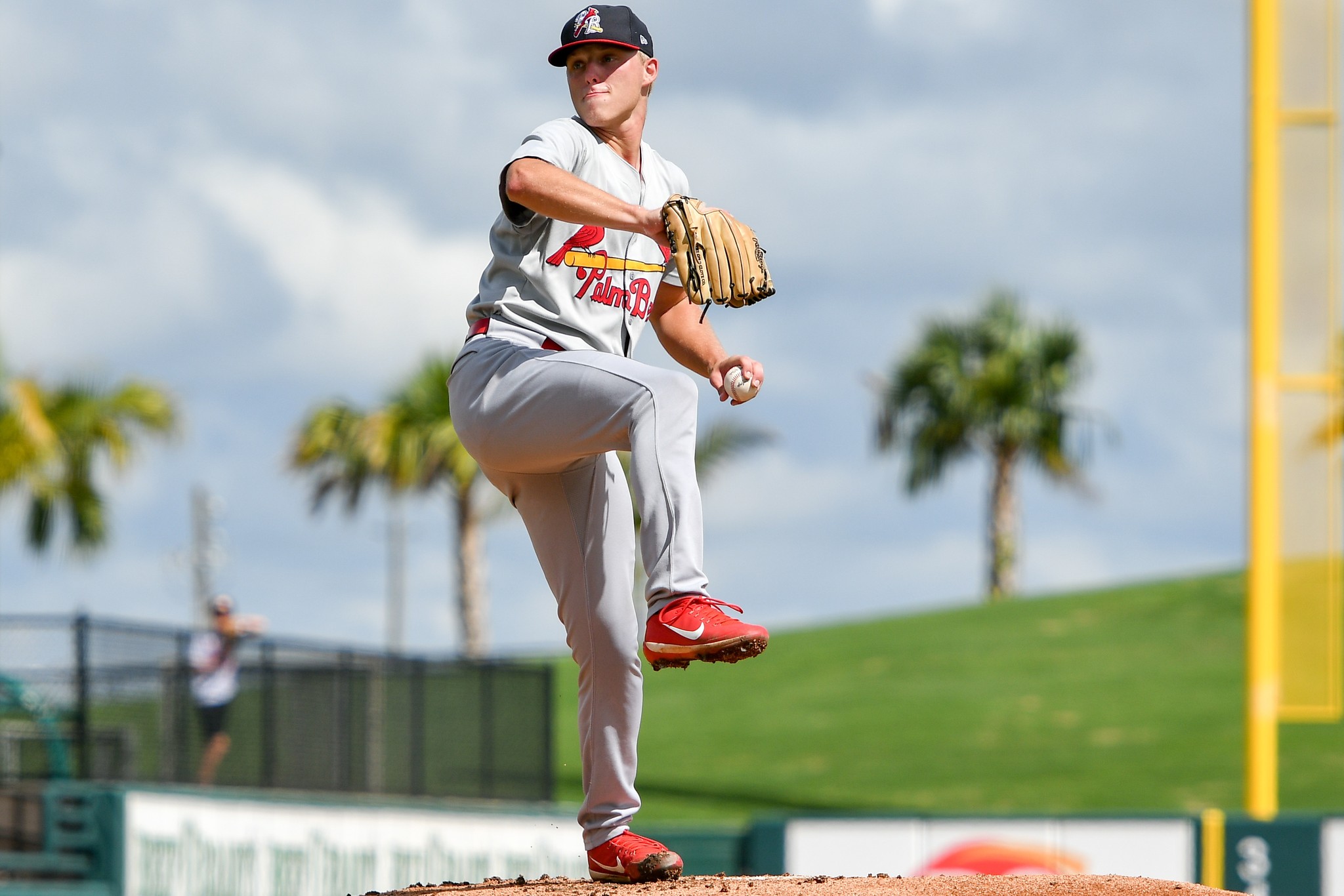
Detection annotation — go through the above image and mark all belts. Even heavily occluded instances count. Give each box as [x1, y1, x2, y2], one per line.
[464, 320, 566, 352]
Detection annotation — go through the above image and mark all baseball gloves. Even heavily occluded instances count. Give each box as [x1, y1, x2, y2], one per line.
[661, 192, 778, 329]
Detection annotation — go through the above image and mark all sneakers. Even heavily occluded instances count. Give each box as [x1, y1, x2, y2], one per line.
[642, 597, 768, 670]
[587, 831, 683, 883]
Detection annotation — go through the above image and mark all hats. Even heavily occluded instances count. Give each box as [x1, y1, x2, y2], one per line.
[210, 596, 232, 615]
[549, 4, 654, 62]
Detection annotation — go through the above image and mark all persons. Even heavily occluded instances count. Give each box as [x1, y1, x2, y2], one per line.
[185, 593, 268, 785]
[445, 4, 776, 884]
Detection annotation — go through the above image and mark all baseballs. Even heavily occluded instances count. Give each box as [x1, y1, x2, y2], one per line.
[723, 365, 761, 402]
